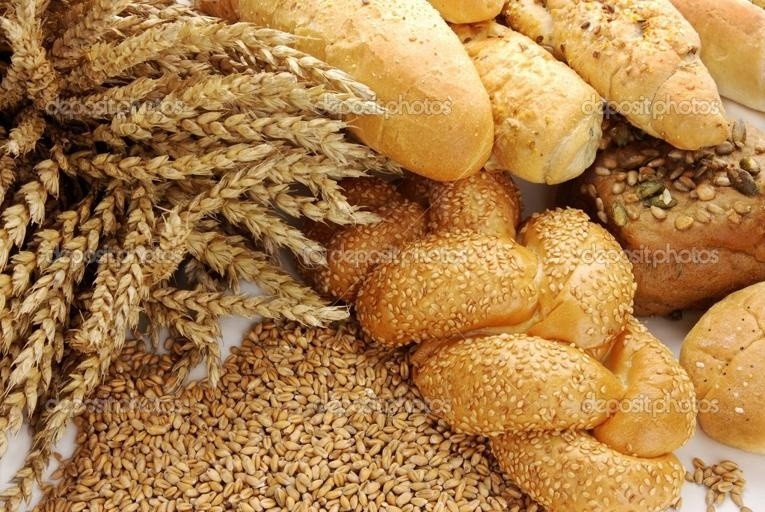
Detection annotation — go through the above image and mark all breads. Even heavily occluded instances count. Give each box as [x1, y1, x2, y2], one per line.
[194, 0, 765, 512]
